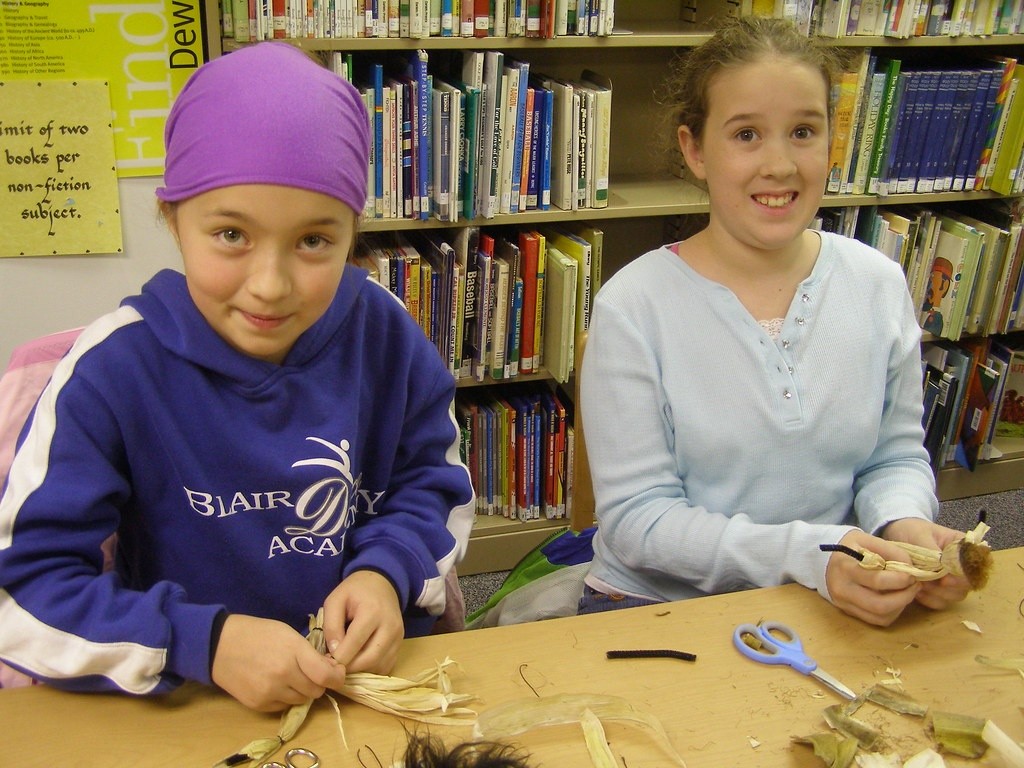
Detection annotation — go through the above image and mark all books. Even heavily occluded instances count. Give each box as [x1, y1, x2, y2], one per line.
[824, 45, 1024, 196]
[919, 342, 1024, 479]
[355, 227, 602, 383]
[454, 388, 574, 523]
[312, 49, 611, 222]
[680, 0, 1024, 39]
[809, 197, 1024, 341]
[221, 0, 615, 40]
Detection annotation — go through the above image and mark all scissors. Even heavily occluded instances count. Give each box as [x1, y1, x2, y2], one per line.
[732, 620, 856, 700]
[262, 747, 322, 768]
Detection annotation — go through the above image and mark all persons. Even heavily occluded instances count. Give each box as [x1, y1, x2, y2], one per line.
[577, 11, 974, 627]
[1, 39, 477, 713]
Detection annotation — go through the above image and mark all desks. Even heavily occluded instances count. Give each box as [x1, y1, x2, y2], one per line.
[0, 546, 1024, 768]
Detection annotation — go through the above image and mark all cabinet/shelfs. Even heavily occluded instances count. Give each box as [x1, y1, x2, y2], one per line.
[202, 0, 1024, 580]
